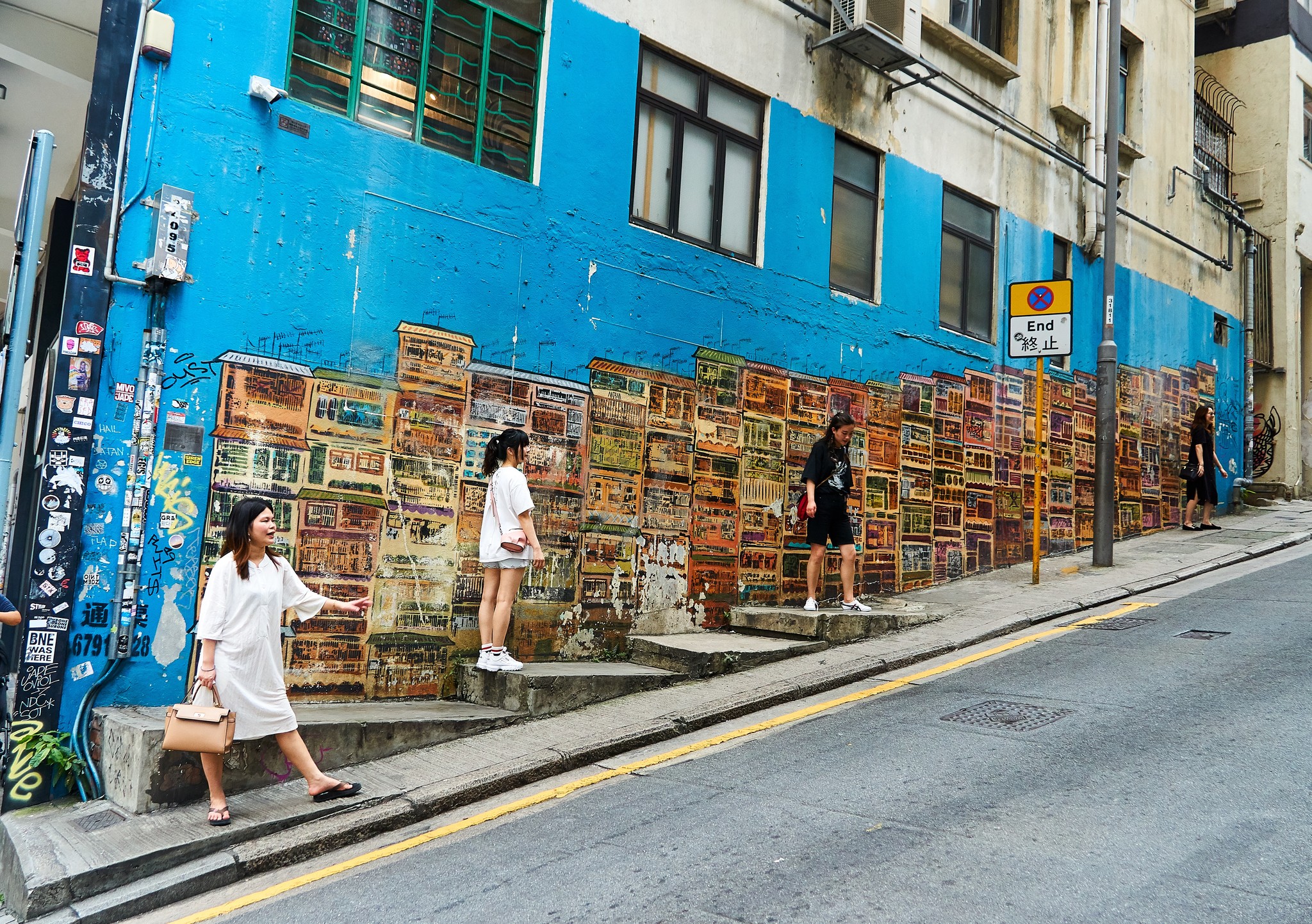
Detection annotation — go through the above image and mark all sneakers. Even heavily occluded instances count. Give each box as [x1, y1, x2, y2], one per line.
[803, 597, 819, 611]
[486, 646, 524, 672]
[476, 650, 490, 669]
[841, 598, 872, 611]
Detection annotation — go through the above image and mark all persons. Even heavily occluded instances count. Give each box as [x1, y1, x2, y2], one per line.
[1182, 405, 1228, 531]
[800, 412, 872, 612]
[191, 497, 373, 826]
[0, 592, 22, 761]
[476, 428, 545, 672]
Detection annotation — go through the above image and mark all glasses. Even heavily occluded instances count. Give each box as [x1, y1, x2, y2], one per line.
[1209, 412, 1216, 415]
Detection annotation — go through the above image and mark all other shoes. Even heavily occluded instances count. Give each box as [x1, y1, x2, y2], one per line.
[1200, 523, 1221, 530]
[1182, 524, 1203, 531]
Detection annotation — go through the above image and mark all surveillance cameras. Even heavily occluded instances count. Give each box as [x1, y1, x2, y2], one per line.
[259, 84, 282, 104]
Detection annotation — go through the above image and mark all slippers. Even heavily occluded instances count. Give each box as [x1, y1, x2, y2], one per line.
[208, 806, 231, 826]
[313, 781, 361, 803]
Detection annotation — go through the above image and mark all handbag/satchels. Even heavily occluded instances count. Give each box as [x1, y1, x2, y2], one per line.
[162, 677, 236, 755]
[797, 491, 809, 523]
[501, 528, 527, 552]
[1179, 464, 1200, 480]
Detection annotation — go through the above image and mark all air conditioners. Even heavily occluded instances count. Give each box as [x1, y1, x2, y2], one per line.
[830, 0, 922, 73]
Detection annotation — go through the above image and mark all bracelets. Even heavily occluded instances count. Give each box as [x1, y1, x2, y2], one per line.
[201, 664, 216, 671]
[1199, 464, 1204, 466]
[333, 600, 338, 610]
